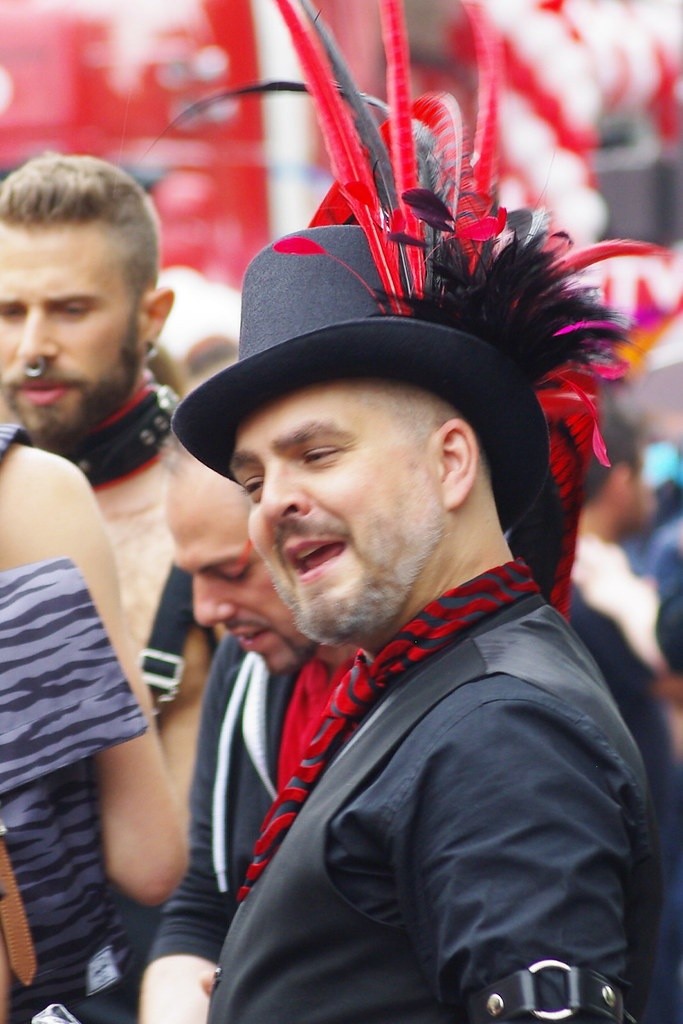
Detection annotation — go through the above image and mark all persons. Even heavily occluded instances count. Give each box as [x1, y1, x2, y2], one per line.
[138, 437, 683, 1024]
[570, 414, 664, 672]
[0, 422, 192, 1024]
[170, 224, 666, 1024]
[0, 153, 229, 728]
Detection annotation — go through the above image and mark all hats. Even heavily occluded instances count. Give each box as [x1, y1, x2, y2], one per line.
[171, 0, 648, 532]
